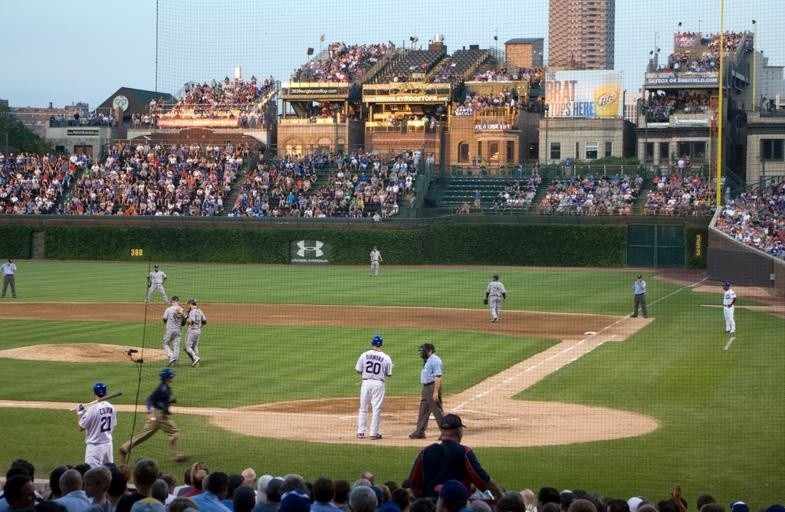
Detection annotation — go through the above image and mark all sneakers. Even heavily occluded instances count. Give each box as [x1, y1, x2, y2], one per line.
[355, 432, 365, 438]
[409, 432, 425, 438]
[369, 434, 382, 440]
[191, 356, 200, 367]
[167, 357, 176, 367]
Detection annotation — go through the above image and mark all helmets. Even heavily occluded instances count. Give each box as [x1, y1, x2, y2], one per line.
[159, 368, 176, 379]
[418, 343, 435, 359]
[722, 281, 730, 286]
[93, 382, 106, 397]
[372, 335, 383, 347]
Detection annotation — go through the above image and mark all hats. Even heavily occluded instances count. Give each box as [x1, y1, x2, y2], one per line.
[627, 497, 644, 512]
[280, 487, 311, 512]
[730, 501, 749, 512]
[440, 414, 466, 430]
[434, 480, 468, 499]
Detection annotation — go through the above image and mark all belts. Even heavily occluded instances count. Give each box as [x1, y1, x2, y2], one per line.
[423, 381, 435, 387]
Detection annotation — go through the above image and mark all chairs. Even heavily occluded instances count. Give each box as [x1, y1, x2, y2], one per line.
[257, 46, 540, 216]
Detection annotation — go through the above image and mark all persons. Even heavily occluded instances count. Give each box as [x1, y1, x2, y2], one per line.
[368, 245, 382, 274]
[458, 156, 726, 217]
[1, 259, 17, 298]
[456, 65, 546, 113]
[641, 31, 748, 122]
[163, 296, 184, 368]
[409, 343, 447, 438]
[475, 119, 512, 132]
[715, 179, 785, 260]
[229, 148, 436, 222]
[630, 275, 649, 319]
[1, 459, 784, 512]
[146, 264, 170, 303]
[286, 40, 456, 128]
[1, 141, 252, 216]
[177, 300, 207, 367]
[76, 383, 118, 469]
[119, 370, 191, 464]
[483, 275, 506, 322]
[353, 336, 392, 439]
[50, 111, 116, 127]
[131, 75, 276, 128]
[721, 280, 736, 333]
[407, 413, 502, 501]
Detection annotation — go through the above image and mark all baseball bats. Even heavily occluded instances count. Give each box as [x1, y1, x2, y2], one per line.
[71, 393, 121, 412]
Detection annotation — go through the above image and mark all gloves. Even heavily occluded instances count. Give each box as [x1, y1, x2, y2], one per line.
[78, 404, 85, 414]
